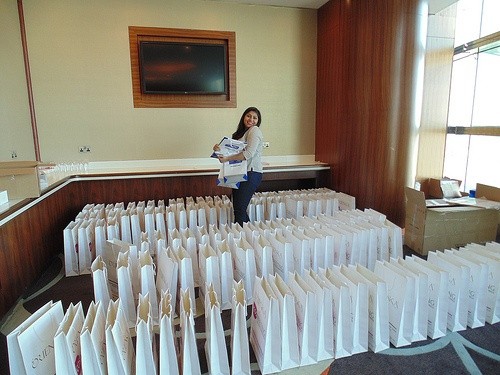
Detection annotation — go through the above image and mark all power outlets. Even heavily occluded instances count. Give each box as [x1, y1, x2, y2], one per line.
[262, 143, 269, 149]
[78, 146, 93, 153]
[10, 150, 18, 159]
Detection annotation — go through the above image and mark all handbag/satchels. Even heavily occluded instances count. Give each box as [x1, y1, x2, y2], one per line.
[159, 288, 179, 375]
[217, 166, 240, 189]
[223, 160, 247, 183]
[135, 292, 158, 375]
[6, 300, 64, 375]
[105, 297, 135, 375]
[54, 301, 85, 375]
[231, 279, 251, 375]
[79, 300, 107, 375]
[250, 240, 500, 375]
[180, 288, 201, 375]
[205, 281, 231, 375]
[210, 136, 247, 162]
[63, 188, 403, 328]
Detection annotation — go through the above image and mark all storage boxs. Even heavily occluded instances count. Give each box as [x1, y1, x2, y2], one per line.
[0, 190, 8, 206]
[404, 176, 500, 256]
[0, 167, 41, 199]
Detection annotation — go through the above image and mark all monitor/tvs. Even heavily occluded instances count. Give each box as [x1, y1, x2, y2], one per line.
[139, 41, 227, 95]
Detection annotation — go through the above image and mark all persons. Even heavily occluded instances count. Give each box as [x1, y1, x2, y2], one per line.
[213, 107, 263, 228]
